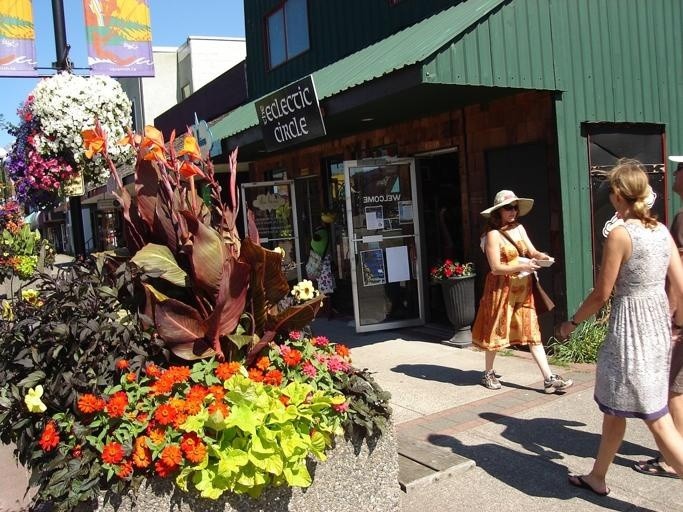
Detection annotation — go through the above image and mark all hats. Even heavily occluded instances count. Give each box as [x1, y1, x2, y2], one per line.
[479, 188, 535, 218]
[667, 155, 683, 163]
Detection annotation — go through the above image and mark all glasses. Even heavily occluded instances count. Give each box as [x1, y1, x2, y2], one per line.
[676, 163, 683, 172]
[502, 204, 521, 212]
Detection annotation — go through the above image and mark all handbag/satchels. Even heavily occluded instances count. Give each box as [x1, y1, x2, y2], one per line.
[534, 280, 557, 316]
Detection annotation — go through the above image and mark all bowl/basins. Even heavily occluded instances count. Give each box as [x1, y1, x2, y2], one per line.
[535, 260, 554, 268]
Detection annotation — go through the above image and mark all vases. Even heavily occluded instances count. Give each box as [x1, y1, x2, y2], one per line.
[435, 270, 479, 348]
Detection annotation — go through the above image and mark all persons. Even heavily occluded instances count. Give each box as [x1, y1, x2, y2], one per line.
[302, 208, 338, 322]
[559, 157, 683, 497]
[633, 152, 682, 480]
[470, 189, 573, 394]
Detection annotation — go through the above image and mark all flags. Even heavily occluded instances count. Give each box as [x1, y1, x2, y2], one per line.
[82, 1, 153, 80]
[0, 1, 38, 79]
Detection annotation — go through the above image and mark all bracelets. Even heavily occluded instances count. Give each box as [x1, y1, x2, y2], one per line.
[671, 320, 682, 331]
[568, 316, 580, 327]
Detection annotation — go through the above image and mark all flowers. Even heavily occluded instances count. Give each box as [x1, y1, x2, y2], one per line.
[1, 72, 390, 510]
[423, 258, 475, 287]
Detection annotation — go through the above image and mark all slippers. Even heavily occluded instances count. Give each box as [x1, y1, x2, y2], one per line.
[567, 473, 611, 497]
[633, 456, 682, 480]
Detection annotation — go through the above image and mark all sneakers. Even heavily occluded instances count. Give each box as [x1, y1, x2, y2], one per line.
[544, 373, 574, 394]
[480, 368, 502, 390]
